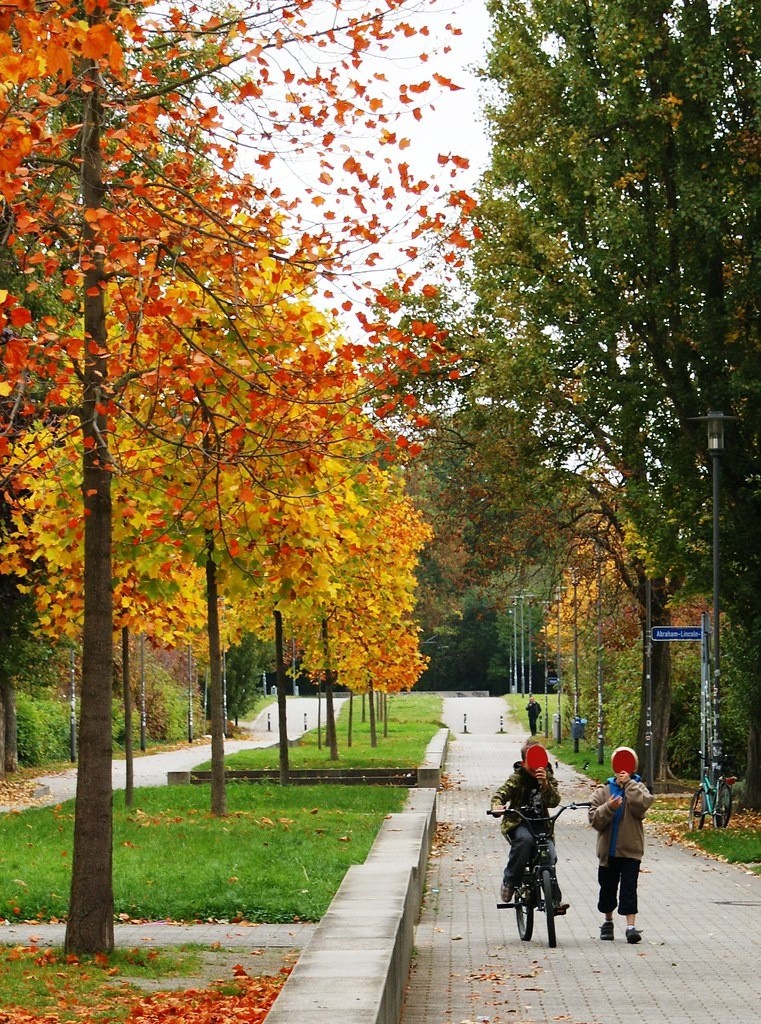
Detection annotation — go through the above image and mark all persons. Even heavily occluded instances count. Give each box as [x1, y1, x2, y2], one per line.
[588, 747, 652, 943]
[526, 697, 542, 736]
[490, 738, 571, 917]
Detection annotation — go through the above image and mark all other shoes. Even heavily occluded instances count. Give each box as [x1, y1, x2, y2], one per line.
[501, 881, 515, 902]
[555, 902, 569, 909]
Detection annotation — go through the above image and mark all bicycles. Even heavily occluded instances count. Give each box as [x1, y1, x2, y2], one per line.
[688, 748, 738, 831]
[485, 801, 591, 948]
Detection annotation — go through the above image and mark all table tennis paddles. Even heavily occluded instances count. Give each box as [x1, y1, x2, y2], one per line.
[611, 749, 636, 788]
[526, 746, 549, 785]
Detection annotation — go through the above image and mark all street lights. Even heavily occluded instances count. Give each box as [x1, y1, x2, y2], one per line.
[686, 408, 742, 757]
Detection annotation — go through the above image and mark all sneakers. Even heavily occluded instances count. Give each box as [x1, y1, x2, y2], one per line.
[626, 928, 641, 943]
[601, 922, 614, 940]
